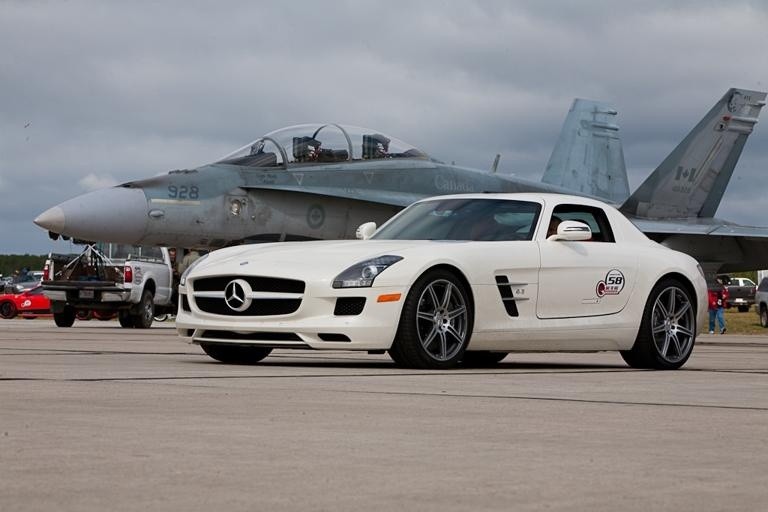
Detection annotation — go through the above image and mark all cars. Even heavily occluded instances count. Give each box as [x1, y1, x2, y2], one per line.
[0, 267, 116, 323]
[718, 275, 767, 328]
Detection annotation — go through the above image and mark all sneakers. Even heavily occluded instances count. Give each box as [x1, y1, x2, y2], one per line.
[710, 331, 714, 334]
[720, 327, 727, 334]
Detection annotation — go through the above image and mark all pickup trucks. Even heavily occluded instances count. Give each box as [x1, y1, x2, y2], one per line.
[38, 241, 208, 331]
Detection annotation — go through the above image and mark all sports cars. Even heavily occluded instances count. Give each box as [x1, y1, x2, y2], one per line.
[169, 188, 710, 374]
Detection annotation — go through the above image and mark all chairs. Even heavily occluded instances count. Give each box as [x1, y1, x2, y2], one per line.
[569, 218, 594, 241]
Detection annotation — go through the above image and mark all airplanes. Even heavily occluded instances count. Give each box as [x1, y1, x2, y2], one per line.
[23, 83, 766, 280]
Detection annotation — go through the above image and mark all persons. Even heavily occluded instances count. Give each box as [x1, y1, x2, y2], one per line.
[708, 278, 729, 334]
[547, 216, 562, 237]
[168, 248, 202, 278]
